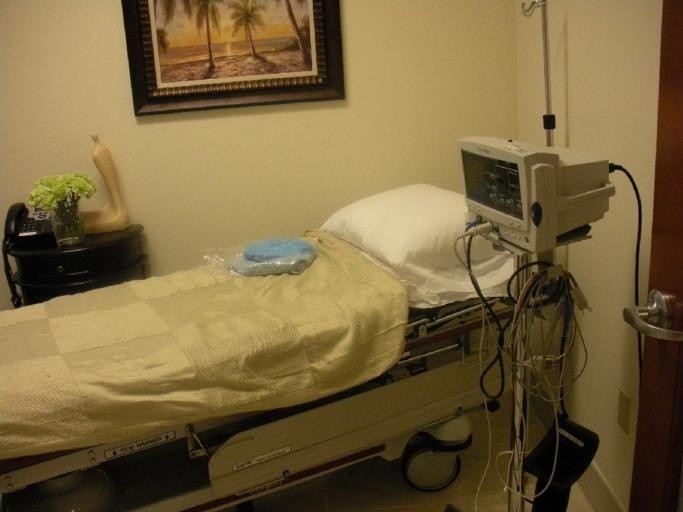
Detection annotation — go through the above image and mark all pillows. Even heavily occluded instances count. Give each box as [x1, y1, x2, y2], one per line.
[318, 182, 513, 306]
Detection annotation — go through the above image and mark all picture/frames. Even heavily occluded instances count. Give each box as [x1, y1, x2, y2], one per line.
[120, 0, 346, 117]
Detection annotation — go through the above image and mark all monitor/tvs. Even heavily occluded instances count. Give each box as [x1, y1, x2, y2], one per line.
[459, 136, 614, 253]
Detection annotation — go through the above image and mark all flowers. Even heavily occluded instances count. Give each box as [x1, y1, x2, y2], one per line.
[26, 172, 97, 230]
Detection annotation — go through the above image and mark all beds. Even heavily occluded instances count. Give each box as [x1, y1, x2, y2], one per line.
[0, 228, 566, 512]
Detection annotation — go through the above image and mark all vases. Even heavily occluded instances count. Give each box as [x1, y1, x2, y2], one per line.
[50, 213, 87, 254]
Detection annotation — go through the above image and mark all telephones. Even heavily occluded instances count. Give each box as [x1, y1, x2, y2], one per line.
[2, 203, 53, 308]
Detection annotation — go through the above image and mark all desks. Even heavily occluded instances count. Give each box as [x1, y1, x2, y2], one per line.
[12, 227, 145, 306]
